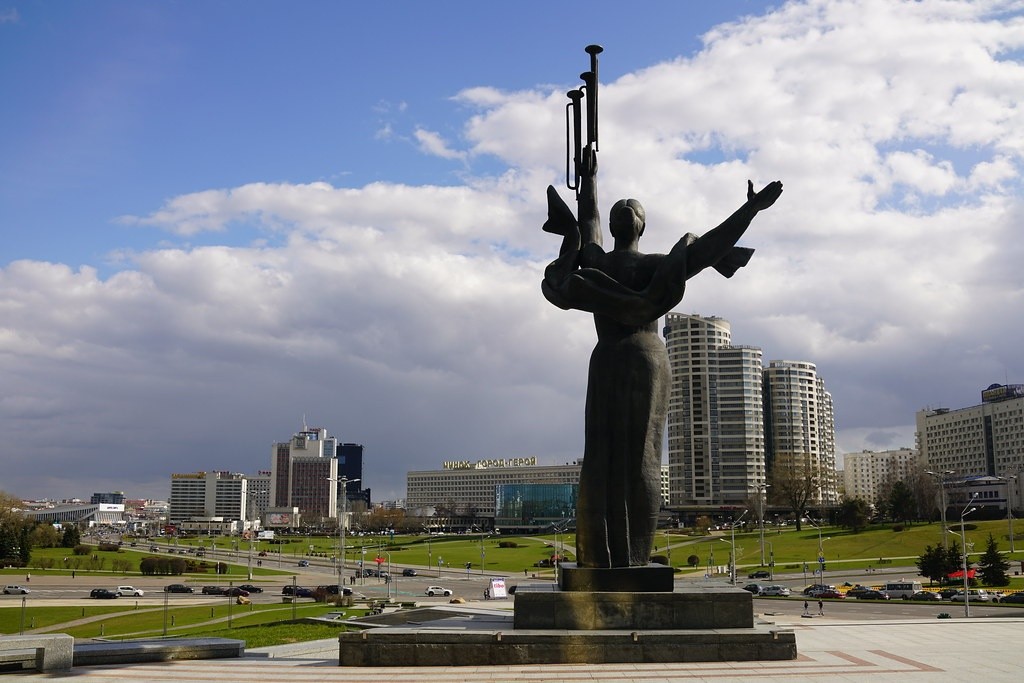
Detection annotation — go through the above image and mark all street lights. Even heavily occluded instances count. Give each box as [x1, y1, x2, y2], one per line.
[325, 476, 361, 605]
[550, 519, 572, 584]
[473, 524, 491, 572]
[925, 470, 956, 552]
[948, 491, 980, 618]
[997, 475, 1018, 553]
[422, 519, 434, 570]
[756, 539, 775, 580]
[748, 483, 771, 566]
[211, 523, 383, 575]
[718, 509, 749, 587]
[806, 514, 831, 573]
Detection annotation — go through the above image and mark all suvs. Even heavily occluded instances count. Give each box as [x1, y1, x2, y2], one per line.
[747, 570, 771, 579]
[424, 585, 453, 597]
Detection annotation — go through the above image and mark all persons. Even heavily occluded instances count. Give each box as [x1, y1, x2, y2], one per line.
[542, 181, 782, 567]
[349, 575, 356, 585]
[71, 570, 75, 579]
[25, 571, 30, 582]
[63, 554, 97, 564]
[803, 598, 824, 615]
[446, 561, 450, 569]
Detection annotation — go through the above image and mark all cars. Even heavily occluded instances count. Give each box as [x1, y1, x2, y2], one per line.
[356, 568, 418, 580]
[89, 584, 355, 599]
[743, 584, 791, 598]
[3, 586, 31, 594]
[298, 560, 310, 568]
[801, 580, 1024, 604]
[80, 527, 208, 558]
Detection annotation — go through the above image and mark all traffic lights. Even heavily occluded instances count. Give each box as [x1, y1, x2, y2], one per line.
[822, 563, 827, 571]
